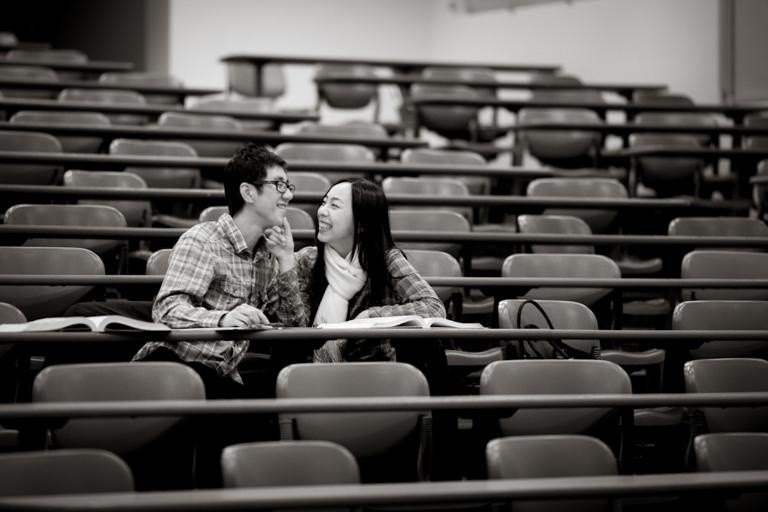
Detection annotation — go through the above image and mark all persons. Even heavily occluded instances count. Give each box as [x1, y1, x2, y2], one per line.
[129, 140, 298, 490]
[260, 174, 462, 482]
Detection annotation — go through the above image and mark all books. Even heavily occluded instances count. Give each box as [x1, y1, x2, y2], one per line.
[312, 313, 485, 334]
[167, 325, 283, 332]
[0, 313, 172, 334]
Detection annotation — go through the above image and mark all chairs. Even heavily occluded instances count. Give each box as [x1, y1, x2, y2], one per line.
[0, 35, 768, 512]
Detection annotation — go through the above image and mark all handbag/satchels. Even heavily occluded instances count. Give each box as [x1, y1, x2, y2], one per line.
[507, 301, 591, 359]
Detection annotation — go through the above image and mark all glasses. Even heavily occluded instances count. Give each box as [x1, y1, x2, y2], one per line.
[253, 181, 295, 193]
